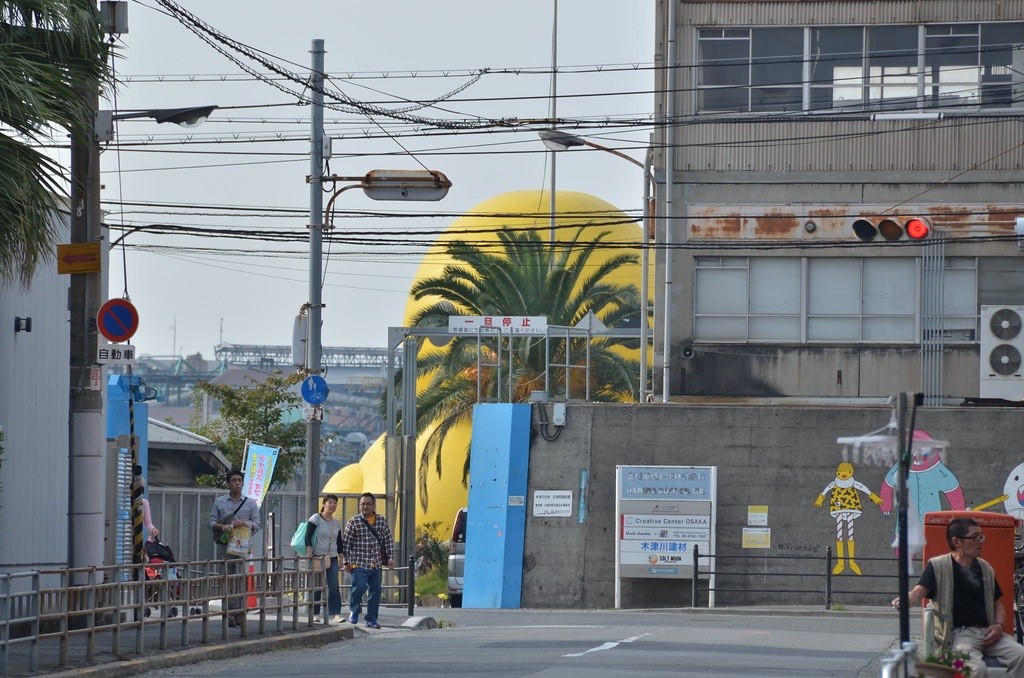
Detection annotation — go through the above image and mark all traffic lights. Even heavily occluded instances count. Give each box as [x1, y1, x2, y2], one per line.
[852, 213, 935, 245]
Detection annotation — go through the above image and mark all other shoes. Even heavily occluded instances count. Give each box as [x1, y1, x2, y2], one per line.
[349, 612, 358, 624]
[366, 620, 381, 629]
[332, 615, 346, 622]
[228, 614, 236, 627]
[314, 614, 320, 622]
[235, 614, 243, 625]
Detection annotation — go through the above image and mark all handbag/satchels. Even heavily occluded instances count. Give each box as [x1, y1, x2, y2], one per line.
[227, 527, 251, 556]
[291, 514, 321, 556]
[212, 514, 235, 544]
[311, 555, 331, 571]
[380, 545, 388, 566]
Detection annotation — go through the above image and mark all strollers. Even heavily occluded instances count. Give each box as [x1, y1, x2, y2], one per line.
[141, 531, 201, 618]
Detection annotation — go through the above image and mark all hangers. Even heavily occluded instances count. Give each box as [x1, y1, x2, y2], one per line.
[837, 395, 950, 467]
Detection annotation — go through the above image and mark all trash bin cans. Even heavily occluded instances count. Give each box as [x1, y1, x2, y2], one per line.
[922, 510, 1015, 637]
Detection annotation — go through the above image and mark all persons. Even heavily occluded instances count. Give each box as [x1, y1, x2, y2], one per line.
[305, 494, 346, 623]
[892, 518, 1024, 678]
[209, 472, 260, 627]
[141, 477, 157, 536]
[341, 493, 395, 629]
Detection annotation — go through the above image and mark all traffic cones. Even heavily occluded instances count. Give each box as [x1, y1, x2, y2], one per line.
[244, 548, 260, 612]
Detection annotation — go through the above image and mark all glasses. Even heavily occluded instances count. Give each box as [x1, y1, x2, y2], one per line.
[957, 534, 985, 542]
[360, 503, 374, 506]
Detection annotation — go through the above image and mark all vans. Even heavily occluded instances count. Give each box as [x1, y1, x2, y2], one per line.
[446, 507, 468, 608]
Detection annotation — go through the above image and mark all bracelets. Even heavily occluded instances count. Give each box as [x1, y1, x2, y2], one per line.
[996, 623, 1004, 632]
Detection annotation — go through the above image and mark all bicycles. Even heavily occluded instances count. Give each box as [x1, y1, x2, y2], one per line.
[1014, 535, 1024, 643]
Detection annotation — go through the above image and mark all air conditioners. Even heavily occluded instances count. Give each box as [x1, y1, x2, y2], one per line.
[979, 304, 1024, 402]
